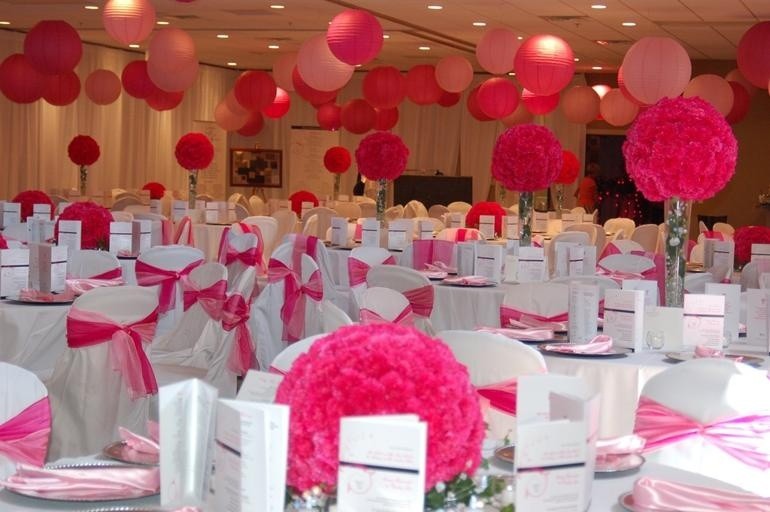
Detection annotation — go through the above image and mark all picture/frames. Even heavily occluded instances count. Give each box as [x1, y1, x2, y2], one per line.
[229, 148, 283, 188]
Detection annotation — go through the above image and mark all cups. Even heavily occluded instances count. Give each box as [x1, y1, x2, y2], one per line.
[645, 328, 665, 349]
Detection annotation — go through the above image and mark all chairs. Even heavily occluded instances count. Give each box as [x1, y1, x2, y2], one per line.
[0, 186, 770, 510]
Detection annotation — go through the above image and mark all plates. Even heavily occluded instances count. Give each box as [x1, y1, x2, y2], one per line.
[494, 439, 647, 474]
[616, 490, 639, 511]
[7, 289, 80, 304]
[103, 438, 164, 465]
[417, 268, 498, 288]
[506, 324, 632, 357]
[3, 462, 162, 504]
[666, 351, 765, 366]
[684, 262, 706, 272]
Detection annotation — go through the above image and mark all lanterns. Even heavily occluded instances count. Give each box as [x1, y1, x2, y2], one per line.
[1, 2, 199, 111]
[215, 9, 770, 133]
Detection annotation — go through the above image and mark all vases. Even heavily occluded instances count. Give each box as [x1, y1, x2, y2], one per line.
[80, 165, 88, 201]
[375, 178, 386, 223]
[332, 174, 342, 205]
[187, 168, 198, 212]
